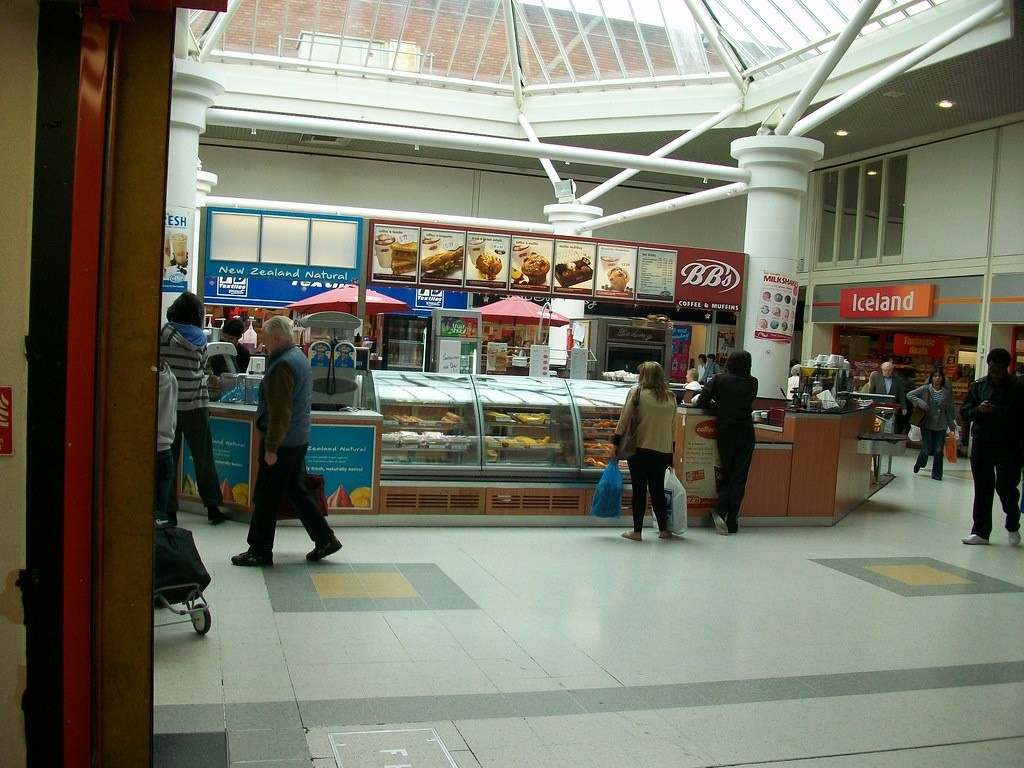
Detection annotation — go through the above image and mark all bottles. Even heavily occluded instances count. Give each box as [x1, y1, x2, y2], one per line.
[812, 374, 822, 397]
[387, 320, 423, 363]
[801, 385, 810, 409]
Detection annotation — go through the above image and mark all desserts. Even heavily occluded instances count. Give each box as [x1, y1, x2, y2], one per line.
[555, 260, 593, 288]
[521, 253, 550, 286]
[607, 267, 630, 291]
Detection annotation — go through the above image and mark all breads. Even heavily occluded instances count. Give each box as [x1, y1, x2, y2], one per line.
[394, 414, 423, 424]
[475, 252, 502, 277]
[442, 411, 459, 422]
[582, 418, 618, 467]
[485, 411, 552, 448]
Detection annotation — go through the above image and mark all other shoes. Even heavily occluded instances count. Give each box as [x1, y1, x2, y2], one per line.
[711, 510, 729, 536]
[932, 475, 942, 480]
[961, 534, 989, 545]
[725, 518, 739, 534]
[913, 463, 920, 473]
[207, 508, 227, 521]
[1008, 531, 1021, 545]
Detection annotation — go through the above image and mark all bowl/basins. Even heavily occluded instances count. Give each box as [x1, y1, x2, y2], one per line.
[809, 401, 821, 411]
[835, 400, 846, 409]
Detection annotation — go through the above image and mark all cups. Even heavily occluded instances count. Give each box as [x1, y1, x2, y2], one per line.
[164, 229, 171, 266]
[468, 238, 486, 265]
[423, 237, 442, 259]
[512, 245, 531, 268]
[806, 354, 849, 368]
[488, 347, 498, 367]
[374, 234, 397, 268]
[170, 233, 188, 264]
[600, 254, 620, 272]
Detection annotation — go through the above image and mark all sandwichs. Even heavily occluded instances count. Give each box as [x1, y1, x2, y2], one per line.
[390, 241, 418, 275]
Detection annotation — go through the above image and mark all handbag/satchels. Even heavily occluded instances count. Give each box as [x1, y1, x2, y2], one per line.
[276, 459, 328, 520]
[651, 466, 688, 534]
[944, 429, 957, 463]
[907, 423, 923, 442]
[616, 388, 641, 460]
[589, 453, 623, 518]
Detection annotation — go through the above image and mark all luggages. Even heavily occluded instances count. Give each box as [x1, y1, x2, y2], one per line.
[152, 515, 212, 634]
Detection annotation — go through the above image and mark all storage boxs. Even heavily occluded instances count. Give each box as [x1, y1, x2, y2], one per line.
[769, 417, 785, 427]
[770, 409, 784, 418]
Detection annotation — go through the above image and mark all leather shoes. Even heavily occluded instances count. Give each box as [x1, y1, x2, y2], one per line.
[231, 544, 273, 566]
[306, 530, 342, 561]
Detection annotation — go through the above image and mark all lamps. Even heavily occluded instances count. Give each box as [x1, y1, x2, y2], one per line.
[197, 157, 202, 171]
[554, 177, 578, 204]
[757, 105, 783, 135]
[188, 25, 201, 61]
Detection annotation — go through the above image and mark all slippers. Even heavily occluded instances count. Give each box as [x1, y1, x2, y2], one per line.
[659, 531, 673, 539]
[621, 531, 643, 542]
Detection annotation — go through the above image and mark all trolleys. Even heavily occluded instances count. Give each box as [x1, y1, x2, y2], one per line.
[151, 520, 213, 637]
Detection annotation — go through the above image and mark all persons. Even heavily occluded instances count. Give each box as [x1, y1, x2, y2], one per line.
[787, 364, 802, 399]
[206, 319, 250, 383]
[697, 354, 707, 382]
[159, 291, 231, 526]
[789, 358, 799, 377]
[231, 316, 342, 566]
[157, 360, 179, 511]
[700, 354, 720, 383]
[1014, 364, 1024, 513]
[519, 341, 530, 358]
[696, 351, 758, 536]
[503, 335, 513, 356]
[682, 368, 699, 404]
[610, 361, 677, 540]
[959, 348, 1024, 545]
[861, 358, 956, 481]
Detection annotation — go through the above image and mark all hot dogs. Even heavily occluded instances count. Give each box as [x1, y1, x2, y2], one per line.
[421, 246, 464, 278]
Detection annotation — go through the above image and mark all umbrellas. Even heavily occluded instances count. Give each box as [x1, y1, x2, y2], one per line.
[283, 285, 413, 314]
[477, 295, 570, 347]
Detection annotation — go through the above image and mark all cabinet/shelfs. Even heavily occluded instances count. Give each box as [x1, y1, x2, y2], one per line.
[362, 368, 632, 482]
